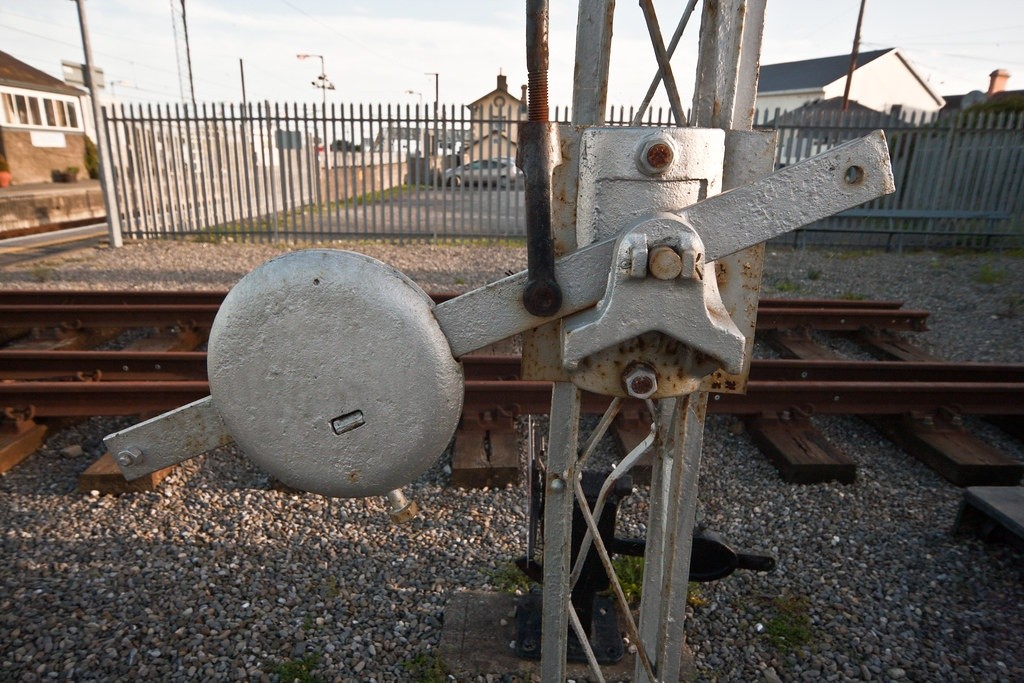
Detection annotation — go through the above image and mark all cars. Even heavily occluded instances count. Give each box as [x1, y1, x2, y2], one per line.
[329, 139, 360, 152]
[444, 155, 524, 191]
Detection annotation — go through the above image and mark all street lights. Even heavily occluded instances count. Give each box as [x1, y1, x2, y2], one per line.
[296, 52, 328, 103]
[423, 72, 440, 102]
